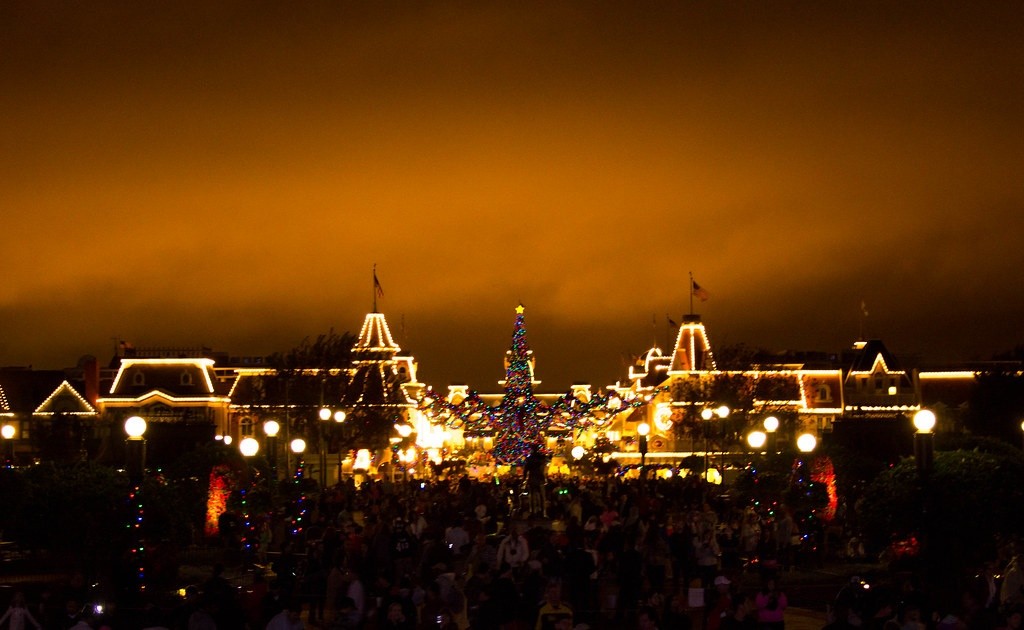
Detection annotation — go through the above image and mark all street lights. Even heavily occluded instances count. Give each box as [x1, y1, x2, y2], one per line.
[1, 425, 19, 463]
[800, 431, 816, 566]
[913, 409, 935, 614]
[238, 439, 260, 570]
[717, 406, 729, 493]
[318, 408, 331, 487]
[291, 438, 308, 549]
[121, 417, 149, 622]
[264, 420, 279, 478]
[765, 416, 779, 476]
[701, 408, 712, 481]
[747, 430, 764, 516]
[398, 425, 412, 499]
[637, 424, 649, 475]
[334, 411, 346, 479]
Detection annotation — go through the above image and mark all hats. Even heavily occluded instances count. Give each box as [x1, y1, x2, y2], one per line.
[714, 576, 732, 585]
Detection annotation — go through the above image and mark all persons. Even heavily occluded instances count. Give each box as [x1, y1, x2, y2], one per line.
[522, 445, 554, 519]
[2, 468, 1024, 630]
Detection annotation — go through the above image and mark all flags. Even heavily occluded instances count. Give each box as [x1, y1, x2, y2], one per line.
[669, 319, 678, 335]
[692, 280, 711, 302]
[861, 301, 869, 316]
[119, 340, 133, 349]
[375, 275, 384, 299]
